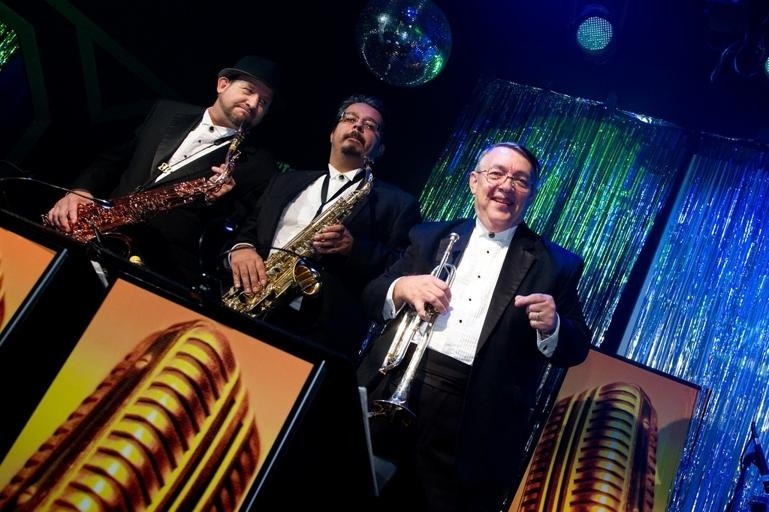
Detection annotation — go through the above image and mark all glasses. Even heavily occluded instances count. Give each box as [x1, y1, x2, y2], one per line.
[474, 167, 533, 194]
[339, 110, 383, 143]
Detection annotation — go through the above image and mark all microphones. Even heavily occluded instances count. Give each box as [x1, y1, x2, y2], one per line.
[0, 317, 262, 512]
[513, 381, 661, 512]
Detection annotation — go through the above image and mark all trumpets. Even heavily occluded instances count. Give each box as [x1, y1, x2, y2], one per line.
[374, 232, 460, 428]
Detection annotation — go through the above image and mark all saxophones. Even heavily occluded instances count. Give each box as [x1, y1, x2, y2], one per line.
[39, 122, 248, 244]
[222, 164, 375, 321]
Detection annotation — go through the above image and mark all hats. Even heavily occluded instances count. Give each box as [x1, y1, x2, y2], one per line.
[220, 56, 286, 99]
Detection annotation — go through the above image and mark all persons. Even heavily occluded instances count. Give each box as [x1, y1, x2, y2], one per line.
[45, 58, 277, 282]
[357, 142, 592, 512]
[203, 93, 420, 354]
